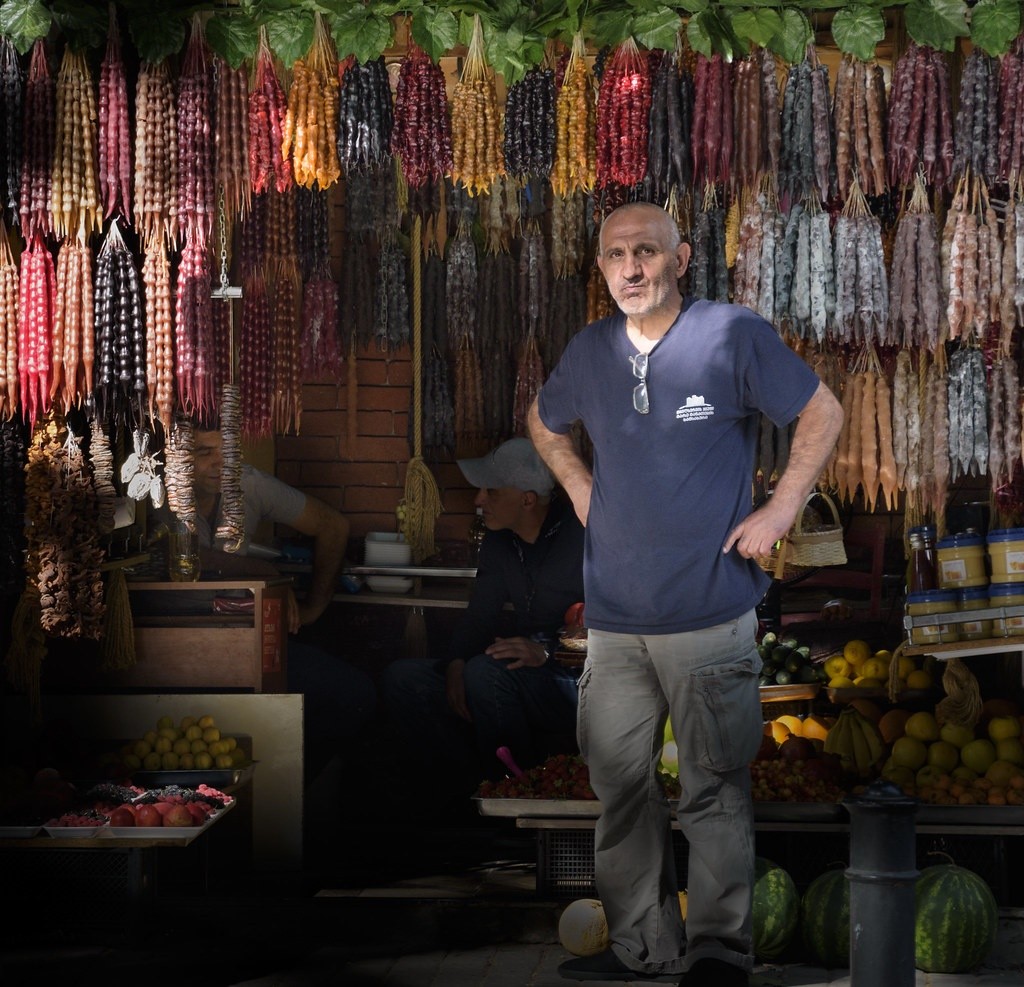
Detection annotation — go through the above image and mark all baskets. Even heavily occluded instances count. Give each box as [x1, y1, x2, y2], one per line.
[754, 492, 847, 574]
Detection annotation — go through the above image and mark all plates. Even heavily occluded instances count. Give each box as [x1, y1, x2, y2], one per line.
[366, 575, 413, 593]
[364, 531, 411, 566]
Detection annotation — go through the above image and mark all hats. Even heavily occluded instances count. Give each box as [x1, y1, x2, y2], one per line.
[456, 437, 555, 496]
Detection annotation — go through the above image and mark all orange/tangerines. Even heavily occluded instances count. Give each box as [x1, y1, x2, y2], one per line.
[762, 700, 909, 741]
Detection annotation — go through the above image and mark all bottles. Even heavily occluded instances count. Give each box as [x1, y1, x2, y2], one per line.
[909, 534, 921, 563]
[467, 507, 486, 567]
[906, 541, 939, 593]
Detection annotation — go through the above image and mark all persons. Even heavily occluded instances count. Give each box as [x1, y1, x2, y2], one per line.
[528, 202, 847, 986]
[127, 410, 377, 864]
[329, 438, 584, 889]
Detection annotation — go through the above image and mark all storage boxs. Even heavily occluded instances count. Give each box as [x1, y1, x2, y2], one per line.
[536, 828, 595, 900]
[760, 682, 823, 722]
[0, 847, 147, 950]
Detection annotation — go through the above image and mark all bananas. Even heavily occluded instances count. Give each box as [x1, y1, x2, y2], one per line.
[823, 705, 885, 774]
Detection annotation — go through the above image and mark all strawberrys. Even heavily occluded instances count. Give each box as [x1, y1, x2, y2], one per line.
[477, 754, 596, 800]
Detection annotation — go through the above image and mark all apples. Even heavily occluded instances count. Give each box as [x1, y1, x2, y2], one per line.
[881, 699, 1024, 788]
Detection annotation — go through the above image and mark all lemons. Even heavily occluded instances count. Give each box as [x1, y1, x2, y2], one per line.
[825, 641, 929, 691]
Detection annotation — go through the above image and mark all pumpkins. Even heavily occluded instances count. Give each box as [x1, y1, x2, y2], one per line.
[677, 890, 688, 923]
[559, 899, 609, 957]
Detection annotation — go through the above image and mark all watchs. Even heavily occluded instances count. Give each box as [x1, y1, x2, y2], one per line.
[543, 644, 551, 660]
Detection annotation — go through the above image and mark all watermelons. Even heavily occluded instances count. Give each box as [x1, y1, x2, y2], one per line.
[746, 857, 998, 973]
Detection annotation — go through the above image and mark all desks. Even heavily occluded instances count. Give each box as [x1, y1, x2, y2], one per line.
[0, 798, 237, 847]
[519, 817, 1024, 836]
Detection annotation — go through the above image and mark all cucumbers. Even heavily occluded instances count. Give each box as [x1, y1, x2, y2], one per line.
[754, 633, 824, 685]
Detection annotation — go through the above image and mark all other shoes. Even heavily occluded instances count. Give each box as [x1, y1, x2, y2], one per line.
[557, 947, 684, 982]
[679, 957, 749, 987]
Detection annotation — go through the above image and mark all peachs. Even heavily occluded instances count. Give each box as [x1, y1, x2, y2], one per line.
[100, 715, 246, 779]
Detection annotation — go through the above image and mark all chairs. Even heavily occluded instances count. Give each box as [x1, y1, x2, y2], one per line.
[780, 521, 886, 627]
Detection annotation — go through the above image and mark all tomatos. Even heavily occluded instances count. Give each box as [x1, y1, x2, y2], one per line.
[109, 801, 206, 827]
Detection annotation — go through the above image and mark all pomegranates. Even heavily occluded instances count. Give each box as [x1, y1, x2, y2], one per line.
[757, 733, 839, 772]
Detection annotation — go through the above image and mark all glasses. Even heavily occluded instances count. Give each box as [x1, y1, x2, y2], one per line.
[628, 352, 650, 414]
